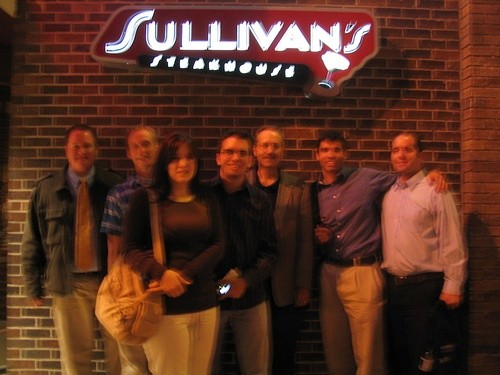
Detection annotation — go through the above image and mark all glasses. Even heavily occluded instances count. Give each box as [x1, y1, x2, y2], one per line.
[220, 150, 251, 158]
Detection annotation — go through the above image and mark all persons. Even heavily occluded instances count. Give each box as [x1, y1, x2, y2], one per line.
[313, 132, 469, 375]
[121, 131, 227, 374]
[311, 130, 449, 375]
[202, 130, 278, 375]
[21, 124, 126, 375]
[245, 125, 313, 375]
[100, 125, 161, 375]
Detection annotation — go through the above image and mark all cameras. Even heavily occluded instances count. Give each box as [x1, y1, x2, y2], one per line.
[217, 269, 241, 295]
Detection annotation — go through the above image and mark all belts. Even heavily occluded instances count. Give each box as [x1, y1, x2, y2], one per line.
[322, 253, 381, 267]
[389, 272, 443, 286]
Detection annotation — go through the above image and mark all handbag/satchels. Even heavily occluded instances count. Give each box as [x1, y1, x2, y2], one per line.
[94, 255, 164, 345]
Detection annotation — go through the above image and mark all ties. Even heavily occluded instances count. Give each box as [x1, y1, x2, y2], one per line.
[75, 179, 91, 272]
